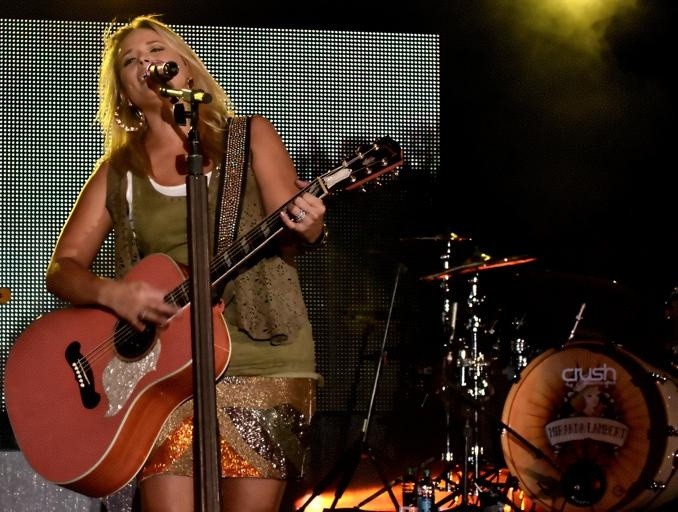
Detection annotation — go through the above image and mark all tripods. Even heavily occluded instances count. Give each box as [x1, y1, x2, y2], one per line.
[298, 258, 404, 512]
[354, 245, 514, 512]
[427, 268, 528, 512]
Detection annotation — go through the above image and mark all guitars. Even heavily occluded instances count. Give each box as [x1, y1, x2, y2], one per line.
[4, 136, 404, 498]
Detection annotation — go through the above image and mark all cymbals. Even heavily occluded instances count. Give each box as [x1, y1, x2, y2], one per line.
[459, 253, 538, 274]
[419, 262, 482, 282]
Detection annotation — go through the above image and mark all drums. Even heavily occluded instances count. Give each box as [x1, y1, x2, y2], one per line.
[503, 336, 547, 375]
[500, 339, 678, 512]
[435, 301, 543, 415]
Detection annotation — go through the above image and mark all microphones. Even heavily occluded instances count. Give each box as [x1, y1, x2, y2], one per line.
[146, 59, 179, 82]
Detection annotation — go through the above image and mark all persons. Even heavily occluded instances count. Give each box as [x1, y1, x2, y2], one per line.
[45, 15, 329, 512]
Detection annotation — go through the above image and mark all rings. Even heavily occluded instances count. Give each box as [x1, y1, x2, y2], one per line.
[140, 310, 147, 318]
[295, 209, 306, 223]
